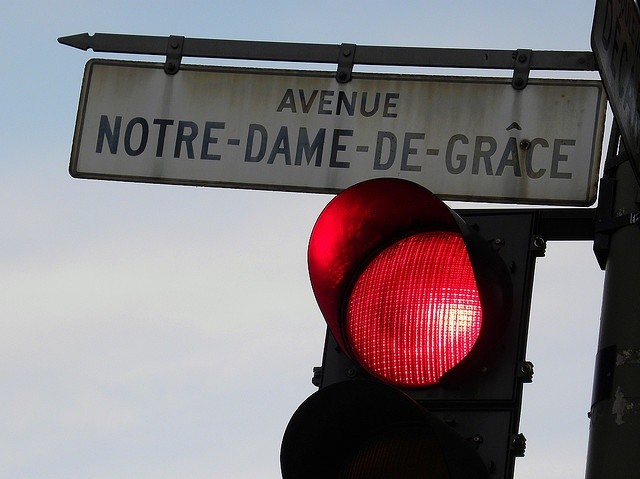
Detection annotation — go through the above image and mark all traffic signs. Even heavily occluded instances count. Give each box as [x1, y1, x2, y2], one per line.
[68, 58, 607, 206]
[590, 0, 640, 189]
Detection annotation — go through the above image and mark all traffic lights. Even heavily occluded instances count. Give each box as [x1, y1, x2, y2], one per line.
[279, 176, 518, 479]
